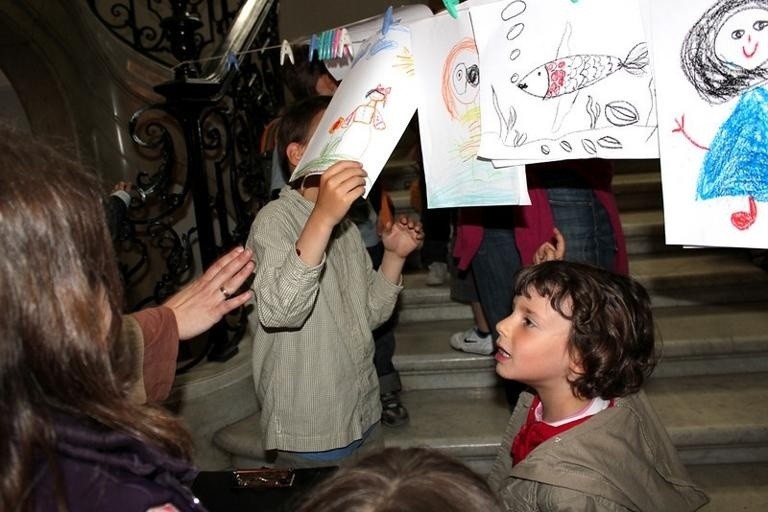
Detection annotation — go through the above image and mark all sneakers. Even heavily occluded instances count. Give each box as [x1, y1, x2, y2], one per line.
[427, 261, 449, 286]
[382, 394, 408, 428]
[450, 327, 495, 355]
